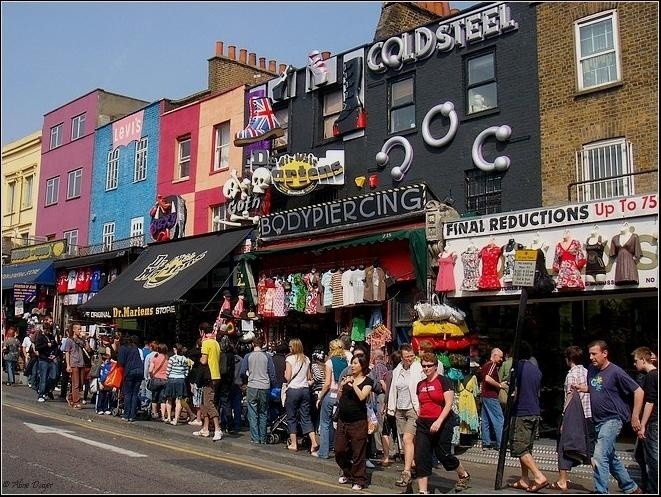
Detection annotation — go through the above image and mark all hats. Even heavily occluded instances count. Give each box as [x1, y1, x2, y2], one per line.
[222, 289, 259, 342]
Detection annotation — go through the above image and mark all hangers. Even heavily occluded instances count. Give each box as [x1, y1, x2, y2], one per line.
[260, 264, 310, 275]
[350, 257, 358, 271]
[373, 255, 379, 267]
[340, 258, 346, 273]
[330, 260, 337, 273]
[359, 257, 365, 270]
[311, 262, 316, 274]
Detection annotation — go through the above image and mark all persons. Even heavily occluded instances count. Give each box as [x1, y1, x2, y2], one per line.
[583, 231, 607, 284]
[387, 343, 422, 487]
[478, 244, 502, 290]
[552, 234, 586, 292]
[609, 226, 642, 285]
[571, 340, 646, 494]
[525, 236, 557, 294]
[501, 239, 523, 284]
[544, 346, 598, 491]
[2, 315, 399, 491]
[415, 351, 471, 494]
[434, 246, 457, 292]
[458, 342, 550, 492]
[629, 347, 658, 494]
[460, 246, 480, 292]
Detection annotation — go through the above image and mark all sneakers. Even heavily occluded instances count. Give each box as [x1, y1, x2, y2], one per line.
[5, 380, 231, 441]
[247, 439, 471, 491]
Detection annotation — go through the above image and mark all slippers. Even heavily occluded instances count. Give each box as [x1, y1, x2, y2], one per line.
[482, 445, 643, 494]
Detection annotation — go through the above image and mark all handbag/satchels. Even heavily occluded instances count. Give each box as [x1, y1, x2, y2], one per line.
[366, 403, 379, 435]
[412, 293, 479, 379]
[220, 352, 234, 383]
[332, 403, 340, 422]
[448, 409, 460, 426]
[281, 382, 289, 408]
[83, 347, 94, 366]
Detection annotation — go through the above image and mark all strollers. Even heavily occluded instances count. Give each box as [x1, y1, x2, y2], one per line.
[266, 393, 305, 448]
